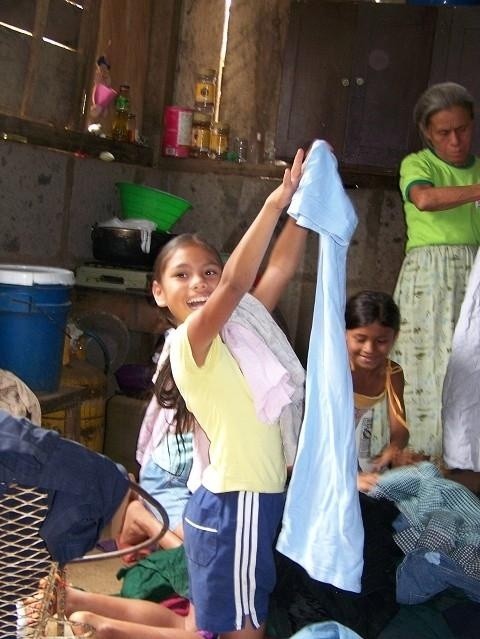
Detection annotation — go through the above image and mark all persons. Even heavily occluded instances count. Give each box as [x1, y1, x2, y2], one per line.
[392, 82, 480, 476]
[40, 147, 312, 638]
[118, 354, 195, 562]
[345, 290, 409, 493]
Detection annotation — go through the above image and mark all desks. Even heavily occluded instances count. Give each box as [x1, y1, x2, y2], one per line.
[65, 284, 175, 337]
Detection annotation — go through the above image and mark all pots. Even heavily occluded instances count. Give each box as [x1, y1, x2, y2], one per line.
[92, 222, 177, 268]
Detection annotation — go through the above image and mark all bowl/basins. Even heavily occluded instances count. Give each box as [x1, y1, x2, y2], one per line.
[114, 180, 194, 235]
[75, 310, 130, 375]
[116, 363, 162, 396]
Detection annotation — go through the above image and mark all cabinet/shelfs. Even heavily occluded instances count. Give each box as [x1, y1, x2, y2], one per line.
[273, 0, 455, 177]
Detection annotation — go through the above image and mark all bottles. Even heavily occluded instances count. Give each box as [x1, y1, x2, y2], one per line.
[113, 84, 131, 144]
[233, 128, 278, 168]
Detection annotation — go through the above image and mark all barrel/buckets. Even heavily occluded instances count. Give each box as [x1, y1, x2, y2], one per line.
[0, 265, 76, 394]
